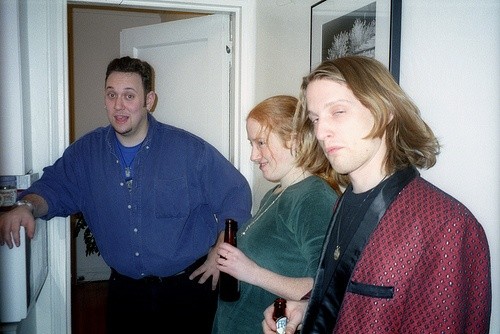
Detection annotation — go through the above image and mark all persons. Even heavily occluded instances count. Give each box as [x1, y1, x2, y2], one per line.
[0, 56, 252, 334]
[260, 57, 492, 334]
[211, 95, 343, 334]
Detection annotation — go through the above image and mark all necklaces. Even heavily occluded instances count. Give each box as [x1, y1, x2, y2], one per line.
[242, 170, 306, 236]
[333, 175, 386, 261]
[115, 132, 146, 178]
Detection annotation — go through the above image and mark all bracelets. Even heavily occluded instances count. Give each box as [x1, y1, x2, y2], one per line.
[11, 199, 36, 216]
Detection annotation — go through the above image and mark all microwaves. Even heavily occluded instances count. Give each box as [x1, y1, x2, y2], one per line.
[0, 212, 48, 323]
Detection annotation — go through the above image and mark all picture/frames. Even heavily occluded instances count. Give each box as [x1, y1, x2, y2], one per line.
[309, 0, 402, 85]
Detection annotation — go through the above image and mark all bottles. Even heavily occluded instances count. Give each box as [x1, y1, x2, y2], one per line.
[218, 218, 241, 302]
[273, 298, 288, 334]
